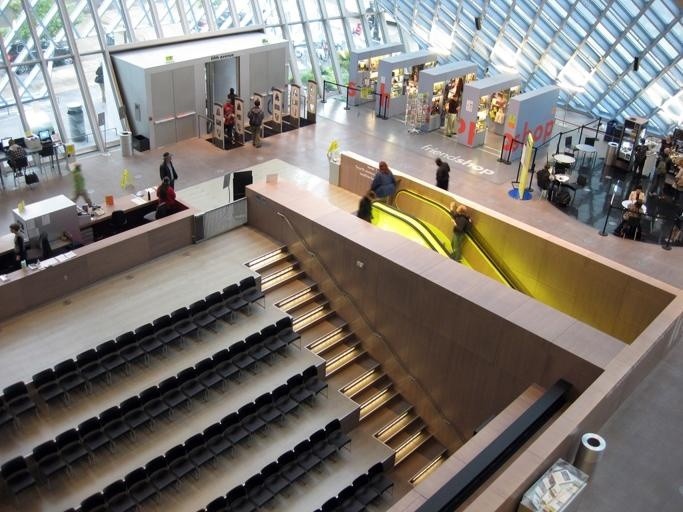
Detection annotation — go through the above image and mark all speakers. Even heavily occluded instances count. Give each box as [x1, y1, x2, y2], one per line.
[475, 17, 481, 30]
[634, 57, 639, 71]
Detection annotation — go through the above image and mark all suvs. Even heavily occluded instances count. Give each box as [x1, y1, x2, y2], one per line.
[0, 35, 74, 75]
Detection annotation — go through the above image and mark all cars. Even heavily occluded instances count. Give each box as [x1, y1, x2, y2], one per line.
[274, 28, 342, 62]
[189, 6, 268, 34]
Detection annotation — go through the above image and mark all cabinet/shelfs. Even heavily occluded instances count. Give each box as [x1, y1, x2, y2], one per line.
[348, 42, 558, 160]
[516, 457, 589, 512]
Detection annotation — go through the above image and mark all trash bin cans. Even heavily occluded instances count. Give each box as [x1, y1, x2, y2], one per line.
[573, 432, 606, 482]
[119, 131, 133, 157]
[605, 142, 618, 167]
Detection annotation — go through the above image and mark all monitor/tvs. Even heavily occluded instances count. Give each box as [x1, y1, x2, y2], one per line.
[1, 137, 12, 148]
[39, 130, 50, 141]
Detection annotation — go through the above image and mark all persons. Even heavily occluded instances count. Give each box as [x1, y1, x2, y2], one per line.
[9, 223, 27, 270]
[7, 140, 24, 177]
[157, 176, 176, 209]
[611, 136, 683, 243]
[223, 99, 235, 149]
[247, 100, 264, 148]
[446, 95, 460, 137]
[435, 158, 450, 191]
[450, 202, 472, 263]
[160, 152, 177, 190]
[70, 165, 92, 207]
[352, 196, 373, 223]
[536, 164, 558, 202]
[227, 88, 237, 105]
[368, 162, 396, 205]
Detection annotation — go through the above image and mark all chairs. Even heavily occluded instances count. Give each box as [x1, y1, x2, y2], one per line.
[0, 128, 170, 263]
[533, 129, 682, 247]
[1, 274, 397, 512]
